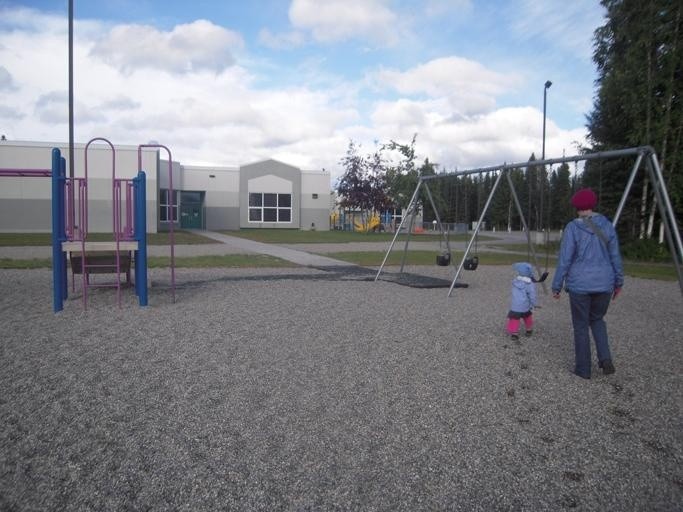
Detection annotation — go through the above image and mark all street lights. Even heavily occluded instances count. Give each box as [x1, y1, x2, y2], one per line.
[538, 82, 553, 231]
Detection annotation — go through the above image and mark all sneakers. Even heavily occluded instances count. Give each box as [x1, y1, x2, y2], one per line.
[525, 329, 533, 338]
[598, 358, 616, 375]
[510, 331, 521, 343]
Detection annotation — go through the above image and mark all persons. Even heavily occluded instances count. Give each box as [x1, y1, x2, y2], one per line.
[550, 187, 624, 380]
[506, 261, 539, 344]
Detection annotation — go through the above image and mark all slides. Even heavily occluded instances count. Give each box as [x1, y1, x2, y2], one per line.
[354, 218, 378, 232]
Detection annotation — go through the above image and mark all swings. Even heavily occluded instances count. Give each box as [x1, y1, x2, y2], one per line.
[463, 170, 479, 270]
[530, 158, 551, 284]
[435, 174, 453, 266]
[573, 153, 603, 221]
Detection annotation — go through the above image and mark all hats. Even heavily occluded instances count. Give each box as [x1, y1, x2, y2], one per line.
[572, 188, 599, 211]
[518, 262, 532, 278]
